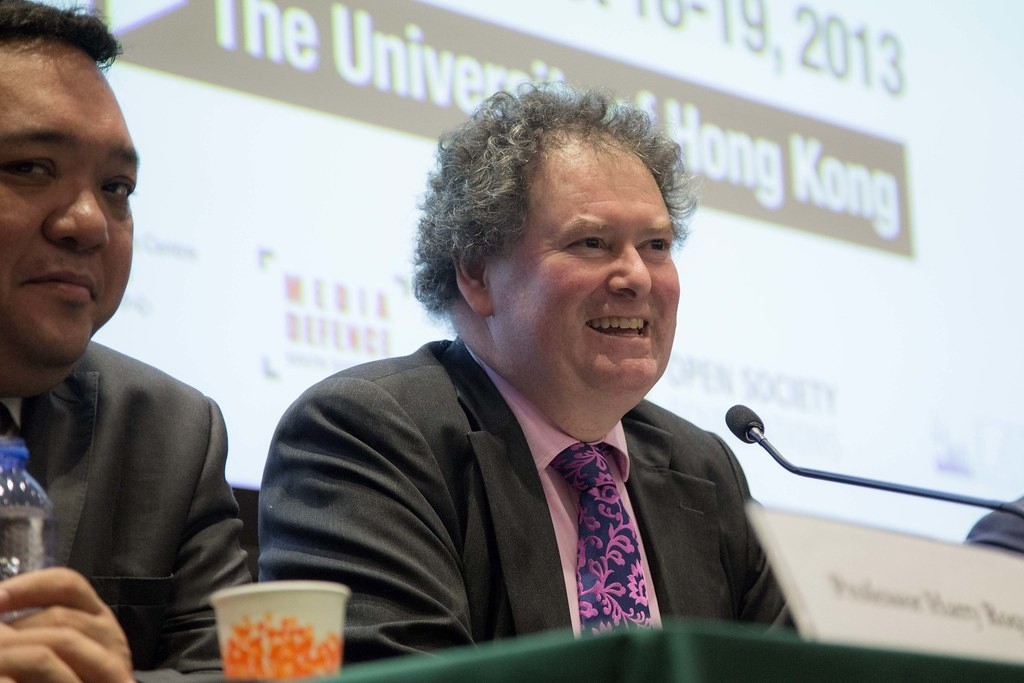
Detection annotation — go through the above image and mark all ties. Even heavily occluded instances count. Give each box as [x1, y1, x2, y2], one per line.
[546, 441, 653, 637]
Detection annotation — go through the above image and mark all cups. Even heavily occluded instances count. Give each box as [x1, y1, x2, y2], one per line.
[208, 578, 353, 682]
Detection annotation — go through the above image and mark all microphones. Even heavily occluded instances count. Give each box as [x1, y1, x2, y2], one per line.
[725, 405, 764, 444]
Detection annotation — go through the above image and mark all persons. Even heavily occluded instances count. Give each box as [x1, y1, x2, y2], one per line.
[0, 0, 257, 683]
[256, 85, 803, 669]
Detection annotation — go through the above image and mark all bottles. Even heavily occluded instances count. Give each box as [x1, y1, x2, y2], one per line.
[0, 433, 60, 628]
